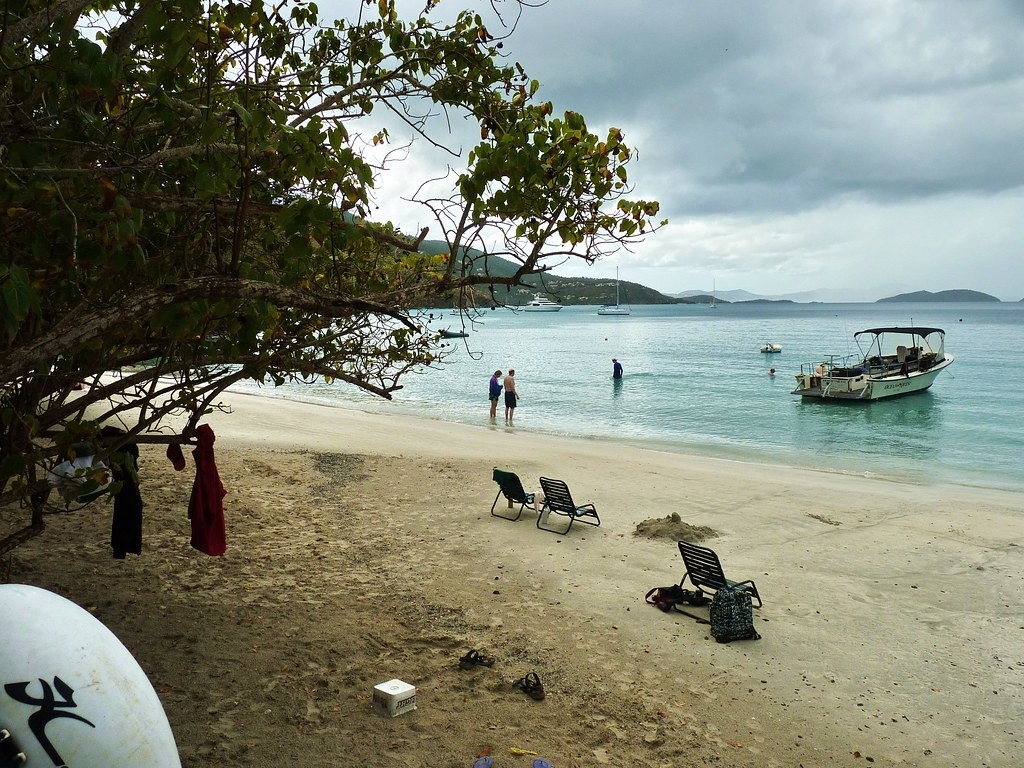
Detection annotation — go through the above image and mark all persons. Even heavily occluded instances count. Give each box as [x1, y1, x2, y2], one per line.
[488, 370, 503, 418]
[504, 368, 519, 420]
[612, 358, 623, 379]
[768, 368, 776, 376]
[815, 364, 827, 377]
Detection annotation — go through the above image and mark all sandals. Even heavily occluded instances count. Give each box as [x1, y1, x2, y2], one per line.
[512, 672, 545, 701]
[459, 650, 496, 669]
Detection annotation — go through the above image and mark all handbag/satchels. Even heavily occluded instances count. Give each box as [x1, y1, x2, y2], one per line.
[645, 584, 684, 613]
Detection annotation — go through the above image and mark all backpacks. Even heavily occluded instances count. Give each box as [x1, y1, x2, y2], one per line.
[710, 588, 761, 644]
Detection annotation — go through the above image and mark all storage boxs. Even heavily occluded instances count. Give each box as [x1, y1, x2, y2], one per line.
[709, 587, 760, 643]
[372, 677, 417, 718]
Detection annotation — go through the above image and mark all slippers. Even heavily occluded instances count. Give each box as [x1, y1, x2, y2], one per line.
[473, 756, 494, 768]
[533, 759, 551, 768]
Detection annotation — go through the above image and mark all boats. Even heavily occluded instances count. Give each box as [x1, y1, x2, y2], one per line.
[790, 318, 954, 400]
[598, 266, 630, 315]
[523, 294, 563, 312]
[709, 277, 718, 308]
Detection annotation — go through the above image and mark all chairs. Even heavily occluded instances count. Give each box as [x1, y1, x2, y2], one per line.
[670, 540, 762, 628]
[536, 476, 601, 536]
[491, 468, 552, 521]
